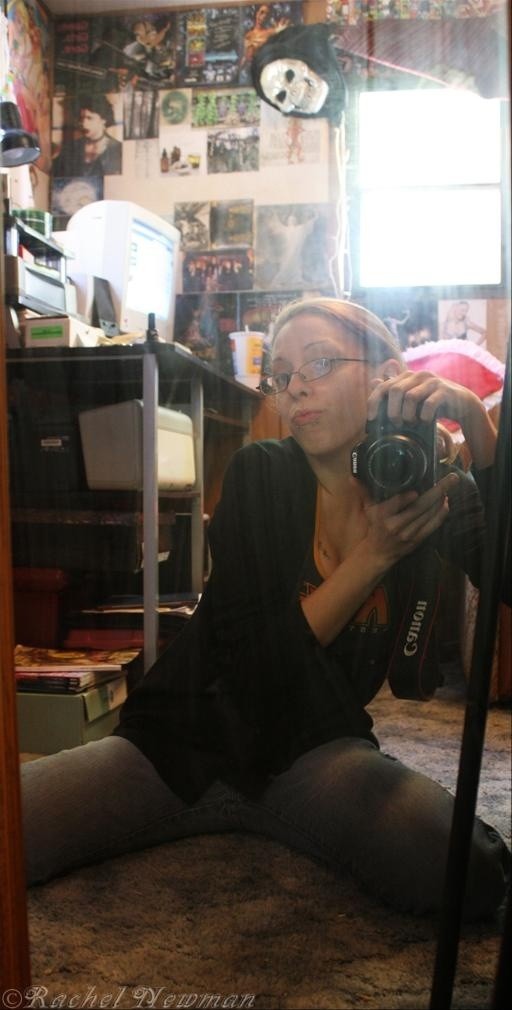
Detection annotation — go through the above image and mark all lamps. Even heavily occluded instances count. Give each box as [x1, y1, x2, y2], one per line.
[0, 100, 42, 167]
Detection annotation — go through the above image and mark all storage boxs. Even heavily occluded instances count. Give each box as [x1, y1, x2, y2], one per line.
[17, 678, 128, 755]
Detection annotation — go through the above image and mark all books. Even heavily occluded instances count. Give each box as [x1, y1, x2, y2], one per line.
[13, 643, 144, 694]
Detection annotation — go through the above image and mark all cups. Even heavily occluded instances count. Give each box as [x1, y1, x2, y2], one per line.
[226, 330, 267, 392]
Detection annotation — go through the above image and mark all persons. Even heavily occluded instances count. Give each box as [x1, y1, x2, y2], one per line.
[240, 4, 295, 74]
[381, 308, 412, 349]
[282, 115, 306, 166]
[185, 250, 255, 294]
[53, 91, 123, 176]
[8, 297, 512, 933]
[438, 299, 487, 350]
[271, 208, 321, 289]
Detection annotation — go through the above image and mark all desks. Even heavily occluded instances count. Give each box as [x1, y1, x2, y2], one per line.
[7, 312, 265, 690]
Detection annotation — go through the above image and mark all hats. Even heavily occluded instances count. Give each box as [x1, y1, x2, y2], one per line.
[54, 95, 115, 124]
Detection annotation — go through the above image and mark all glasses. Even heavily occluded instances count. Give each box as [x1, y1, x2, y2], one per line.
[254, 355, 370, 396]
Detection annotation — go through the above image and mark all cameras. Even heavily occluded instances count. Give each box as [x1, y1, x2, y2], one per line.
[351, 401, 438, 512]
